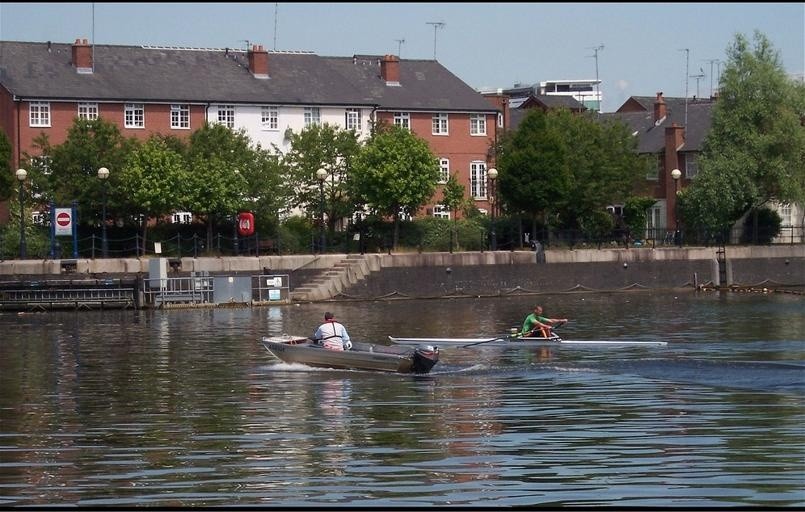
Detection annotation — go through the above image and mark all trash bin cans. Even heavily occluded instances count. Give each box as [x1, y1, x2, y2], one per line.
[484, 230, 497, 250]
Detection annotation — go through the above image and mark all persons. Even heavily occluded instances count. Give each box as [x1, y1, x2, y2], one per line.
[521, 303, 568, 338]
[314, 310, 351, 350]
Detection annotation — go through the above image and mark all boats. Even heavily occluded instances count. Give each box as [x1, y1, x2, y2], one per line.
[389, 335, 668, 351]
[261, 333, 439, 374]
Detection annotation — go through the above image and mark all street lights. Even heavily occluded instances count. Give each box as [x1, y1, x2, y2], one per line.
[315, 168, 328, 254]
[672, 169, 682, 246]
[488, 169, 498, 250]
[16, 168, 28, 259]
[97, 167, 110, 257]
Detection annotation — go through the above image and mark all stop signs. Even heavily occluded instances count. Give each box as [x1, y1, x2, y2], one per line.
[54, 207, 73, 237]
